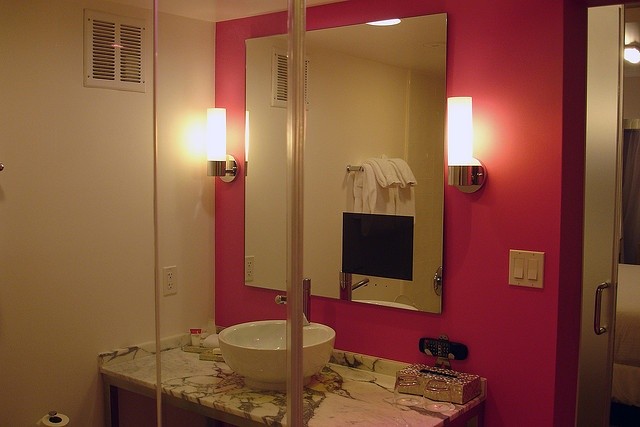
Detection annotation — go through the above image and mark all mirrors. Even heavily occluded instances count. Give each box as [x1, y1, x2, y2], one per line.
[244, 13, 449, 315]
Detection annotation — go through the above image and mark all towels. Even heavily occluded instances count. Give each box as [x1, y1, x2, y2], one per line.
[387, 157, 419, 217]
[354, 159, 399, 215]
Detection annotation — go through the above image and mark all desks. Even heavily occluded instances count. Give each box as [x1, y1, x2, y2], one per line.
[98, 328, 487, 427]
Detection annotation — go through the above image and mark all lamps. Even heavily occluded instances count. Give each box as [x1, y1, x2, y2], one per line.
[446, 95, 487, 193]
[245, 109, 250, 177]
[206, 108, 238, 183]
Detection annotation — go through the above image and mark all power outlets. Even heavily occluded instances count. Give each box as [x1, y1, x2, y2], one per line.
[164, 265, 176, 296]
[246, 256, 255, 284]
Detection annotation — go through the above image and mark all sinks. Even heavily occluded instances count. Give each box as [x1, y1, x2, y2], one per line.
[215, 318, 336, 390]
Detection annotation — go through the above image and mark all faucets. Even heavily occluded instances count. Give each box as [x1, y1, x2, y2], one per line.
[275, 275, 314, 324]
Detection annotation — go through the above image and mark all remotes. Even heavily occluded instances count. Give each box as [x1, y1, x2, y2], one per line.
[419, 338, 468, 360]
